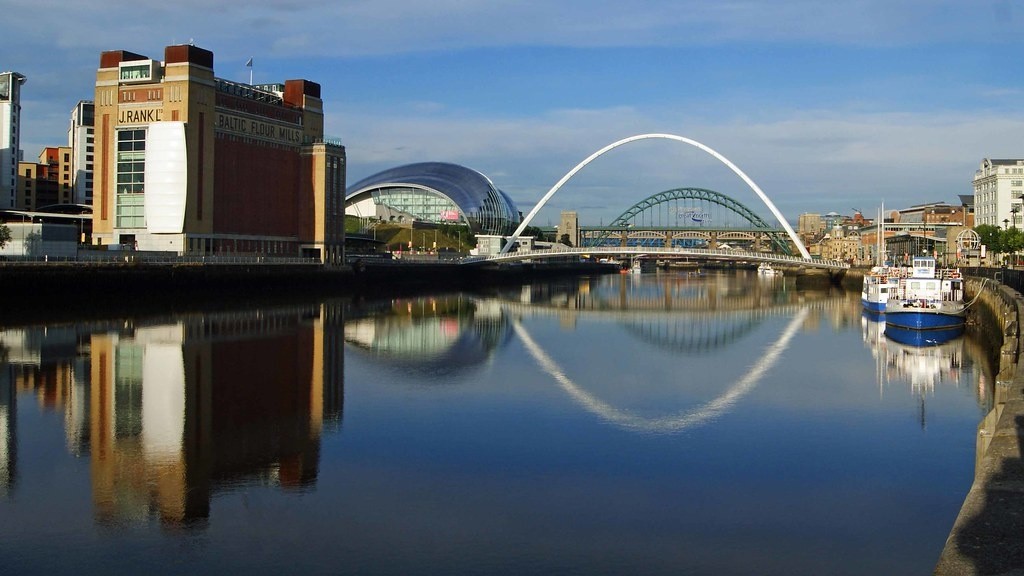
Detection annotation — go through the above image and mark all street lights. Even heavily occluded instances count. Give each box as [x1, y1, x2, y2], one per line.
[1011, 208, 1019, 270]
[1002, 219, 1010, 266]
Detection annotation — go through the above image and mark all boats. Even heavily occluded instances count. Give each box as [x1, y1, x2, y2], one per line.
[884, 324, 965, 432]
[861, 201, 900, 313]
[883, 220, 965, 329]
[861, 308, 900, 399]
